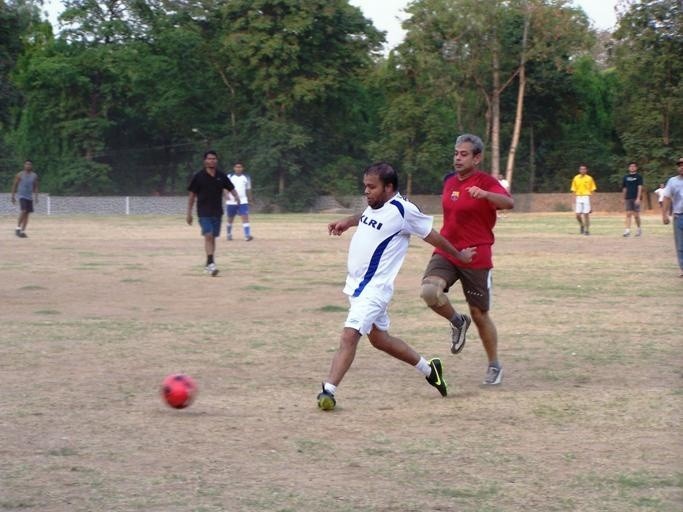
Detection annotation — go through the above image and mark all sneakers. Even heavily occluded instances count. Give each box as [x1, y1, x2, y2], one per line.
[245, 236, 252, 241]
[316, 389, 336, 411]
[624, 229, 631, 236]
[208, 263, 219, 275]
[449, 312, 472, 355]
[482, 362, 503, 386]
[425, 357, 449, 397]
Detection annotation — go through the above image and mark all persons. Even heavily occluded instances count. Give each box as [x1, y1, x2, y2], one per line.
[570, 166, 597, 235]
[420, 134, 514, 389]
[663, 157, 682, 278]
[222, 162, 254, 244]
[316, 163, 477, 409]
[654, 182, 665, 208]
[618, 162, 645, 236]
[183, 151, 243, 277]
[10, 158, 38, 238]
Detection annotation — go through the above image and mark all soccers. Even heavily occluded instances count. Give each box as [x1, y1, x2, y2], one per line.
[163, 373, 195, 407]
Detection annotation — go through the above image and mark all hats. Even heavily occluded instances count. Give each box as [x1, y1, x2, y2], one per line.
[676, 157, 683, 165]
